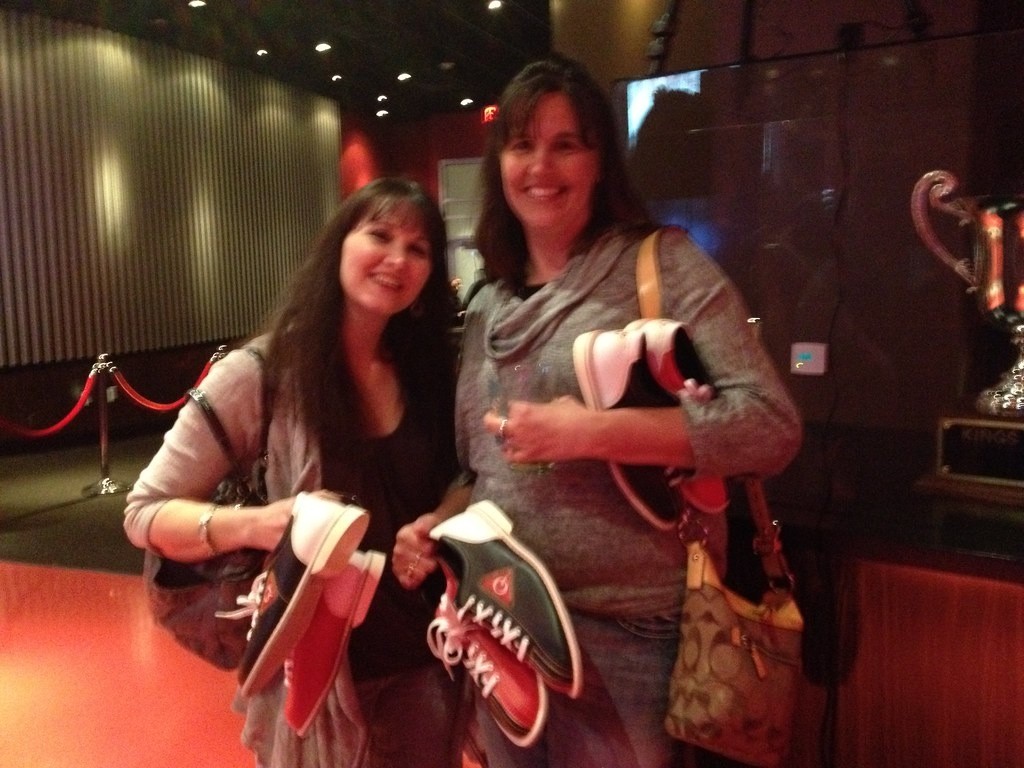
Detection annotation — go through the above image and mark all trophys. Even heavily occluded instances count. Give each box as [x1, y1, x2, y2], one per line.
[912, 168, 1024, 417]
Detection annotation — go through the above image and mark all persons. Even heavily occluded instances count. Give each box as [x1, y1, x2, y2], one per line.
[392, 44, 806, 767]
[122, 173, 463, 767]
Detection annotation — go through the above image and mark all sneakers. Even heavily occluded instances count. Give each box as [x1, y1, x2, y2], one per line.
[571, 328, 692, 529]
[624, 318, 729, 514]
[214, 490, 371, 700]
[280, 551, 387, 740]
[429, 499, 583, 700]
[402, 554, 552, 749]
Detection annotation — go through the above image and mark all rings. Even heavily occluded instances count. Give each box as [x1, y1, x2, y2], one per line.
[408, 563, 415, 576]
[415, 552, 422, 566]
[494, 419, 507, 443]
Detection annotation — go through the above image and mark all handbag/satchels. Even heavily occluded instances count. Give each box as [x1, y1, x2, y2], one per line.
[142, 346, 274, 669]
[665, 541, 803, 767]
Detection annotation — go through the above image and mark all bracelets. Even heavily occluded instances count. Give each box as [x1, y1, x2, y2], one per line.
[199, 502, 217, 555]
